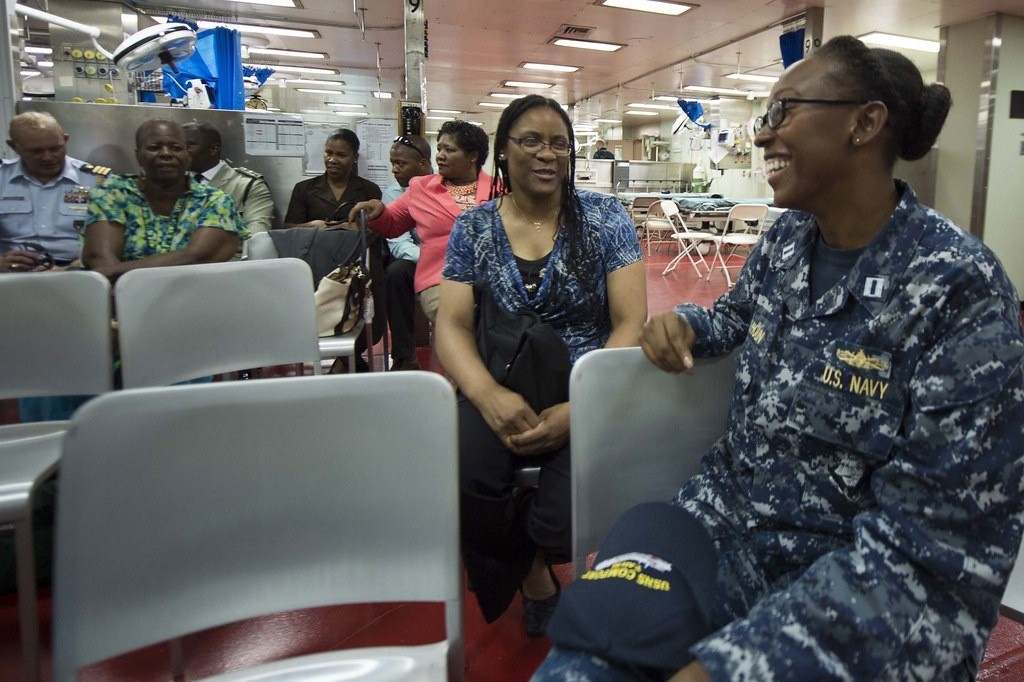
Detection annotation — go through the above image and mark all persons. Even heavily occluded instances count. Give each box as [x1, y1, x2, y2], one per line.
[64, 118, 240, 397]
[593, 140, 614, 161]
[521, 36, 1023, 681]
[345, 121, 508, 399]
[433, 94, 647, 641]
[382, 135, 440, 371]
[182, 121, 276, 380]
[0, 111, 113, 425]
[282, 129, 392, 374]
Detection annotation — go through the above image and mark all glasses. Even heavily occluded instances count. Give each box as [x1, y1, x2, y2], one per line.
[393, 136, 425, 159]
[753, 96, 870, 135]
[507, 133, 572, 156]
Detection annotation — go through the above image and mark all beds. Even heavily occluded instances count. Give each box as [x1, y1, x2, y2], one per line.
[611, 178, 788, 239]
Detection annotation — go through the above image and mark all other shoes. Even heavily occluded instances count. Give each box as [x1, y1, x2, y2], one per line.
[518, 560, 561, 642]
[389, 355, 422, 372]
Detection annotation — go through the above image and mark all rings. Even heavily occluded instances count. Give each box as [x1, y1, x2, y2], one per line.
[10, 263, 19, 269]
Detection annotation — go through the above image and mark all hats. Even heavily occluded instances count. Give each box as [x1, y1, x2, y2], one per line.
[546, 501, 725, 679]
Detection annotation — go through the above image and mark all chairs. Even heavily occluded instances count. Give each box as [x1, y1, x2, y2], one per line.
[0, 196, 770, 682]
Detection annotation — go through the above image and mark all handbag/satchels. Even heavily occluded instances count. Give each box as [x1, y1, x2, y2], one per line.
[469, 285, 573, 416]
[313, 252, 367, 338]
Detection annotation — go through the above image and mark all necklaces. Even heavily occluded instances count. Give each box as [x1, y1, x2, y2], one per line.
[442, 177, 478, 196]
[510, 191, 562, 233]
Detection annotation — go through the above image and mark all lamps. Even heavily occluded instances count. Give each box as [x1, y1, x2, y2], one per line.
[245, 98, 268, 110]
